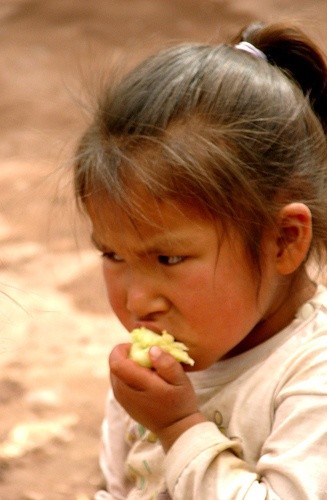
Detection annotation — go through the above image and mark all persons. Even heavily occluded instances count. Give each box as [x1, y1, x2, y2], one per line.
[74, 20, 325, 500]
